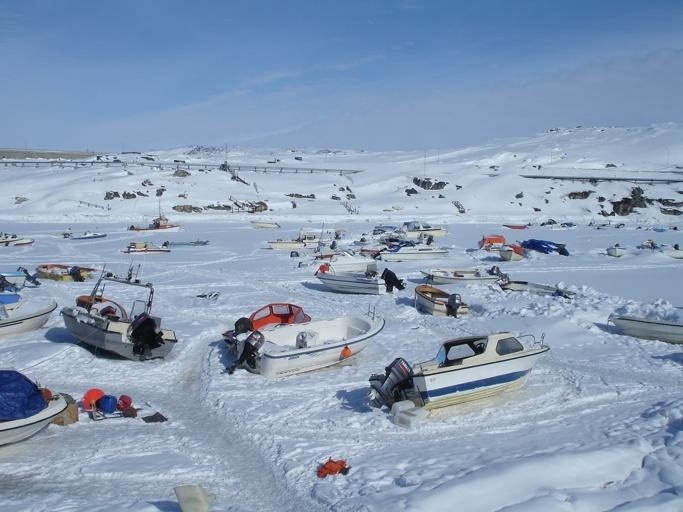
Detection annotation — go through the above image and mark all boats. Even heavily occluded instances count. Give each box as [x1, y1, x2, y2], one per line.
[36, 264, 109, 283]
[0, 369, 68, 446]
[0, 294, 57, 335]
[221, 303, 310, 357]
[419, 266, 510, 284]
[607, 313, 682, 344]
[414, 274, 468, 319]
[225, 303, 385, 378]
[316, 262, 406, 295]
[59, 259, 177, 362]
[499, 281, 576, 305]
[368, 331, 550, 411]
